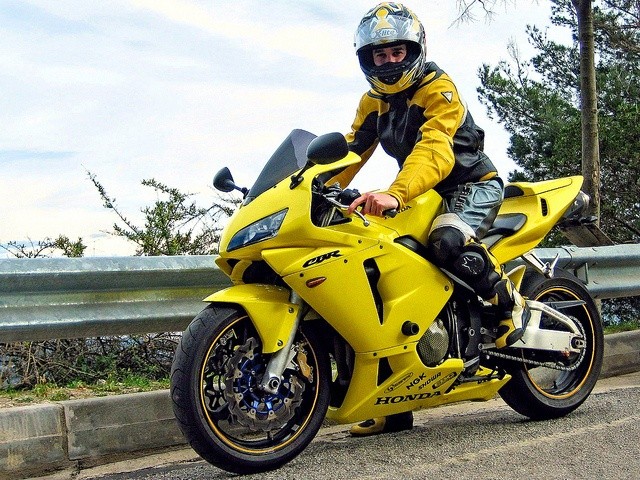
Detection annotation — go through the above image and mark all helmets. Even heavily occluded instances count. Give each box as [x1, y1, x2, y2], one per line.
[354, 2, 426, 93]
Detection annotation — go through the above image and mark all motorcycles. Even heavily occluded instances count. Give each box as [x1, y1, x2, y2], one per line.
[169, 129, 604, 473]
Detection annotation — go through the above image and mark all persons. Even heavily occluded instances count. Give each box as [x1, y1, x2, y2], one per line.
[318, 0, 532, 434]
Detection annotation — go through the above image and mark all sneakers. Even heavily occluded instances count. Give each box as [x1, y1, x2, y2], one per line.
[495, 302, 531, 350]
[350, 411, 413, 435]
